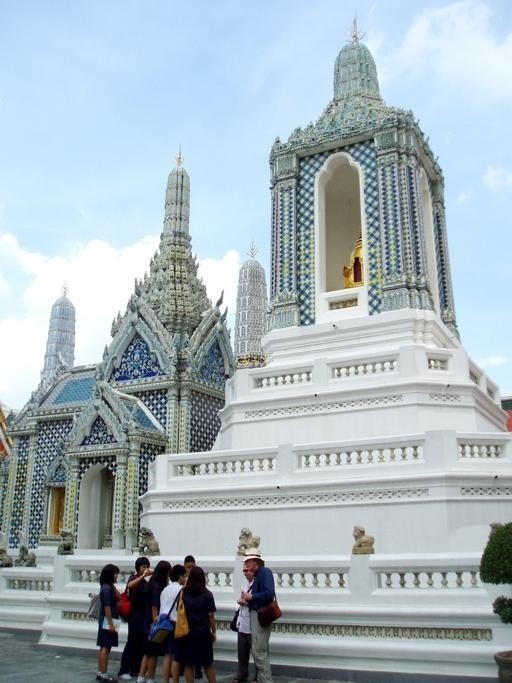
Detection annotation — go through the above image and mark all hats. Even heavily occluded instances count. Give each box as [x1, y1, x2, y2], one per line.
[241, 548, 265, 563]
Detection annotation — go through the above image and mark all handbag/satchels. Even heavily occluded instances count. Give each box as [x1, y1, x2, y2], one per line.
[118, 581, 137, 622]
[148, 613, 174, 644]
[87, 593, 102, 618]
[174, 589, 190, 641]
[256, 600, 282, 628]
[229, 607, 240, 632]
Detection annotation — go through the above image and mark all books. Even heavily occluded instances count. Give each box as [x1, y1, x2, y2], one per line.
[101, 615, 121, 632]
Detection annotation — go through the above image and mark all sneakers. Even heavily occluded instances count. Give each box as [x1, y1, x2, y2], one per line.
[233, 674, 258, 683]
[136, 676, 154, 683]
[118, 673, 133, 680]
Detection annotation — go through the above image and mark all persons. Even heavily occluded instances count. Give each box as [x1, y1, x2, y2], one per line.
[240, 547, 277, 683]
[96, 563, 122, 683]
[232, 563, 260, 682]
[117, 553, 219, 682]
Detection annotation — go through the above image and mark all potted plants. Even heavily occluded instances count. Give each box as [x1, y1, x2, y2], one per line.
[479, 521, 512, 683]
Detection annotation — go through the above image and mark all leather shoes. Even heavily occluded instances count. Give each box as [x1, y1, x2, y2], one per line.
[96, 675, 119, 683]
